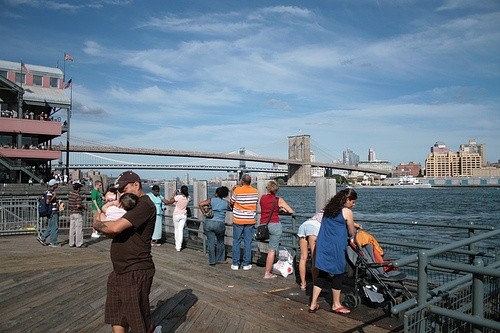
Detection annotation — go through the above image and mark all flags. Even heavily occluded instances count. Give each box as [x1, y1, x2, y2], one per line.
[21, 61, 27, 74]
[64, 53, 72, 61]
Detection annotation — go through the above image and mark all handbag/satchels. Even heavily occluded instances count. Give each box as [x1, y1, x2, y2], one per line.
[204, 198, 214, 218]
[38, 193, 52, 216]
[255, 225, 270, 240]
[187, 207, 192, 217]
[272, 250, 293, 277]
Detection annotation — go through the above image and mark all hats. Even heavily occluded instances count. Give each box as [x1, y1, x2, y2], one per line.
[72, 181, 83, 189]
[48, 179, 59, 186]
[109, 171, 141, 193]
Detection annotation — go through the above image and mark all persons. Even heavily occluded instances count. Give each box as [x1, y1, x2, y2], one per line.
[37, 179, 62, 248]
[228, 174, 259, 270]
[93, 170, 157, 333]
[105, 184, 120, 202]
[259, 181, 293, 278]
[0, 110, 51, 121]
[91, 180, 105, 238]
[309, 188, 362, 315]
[0, 142, 59, 151]
[68, 183, 88, 248]
[297, 209, 325, 289]
[69, 174, 87, 185]
[146, 185, 178, 246]
[169, 185, 190, 251]
[199, 186, 230, 266]
[0, 161, 63, 184]
[97, 193, 139, 222]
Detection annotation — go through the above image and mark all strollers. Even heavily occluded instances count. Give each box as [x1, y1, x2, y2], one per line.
[341, 224, 418, 320]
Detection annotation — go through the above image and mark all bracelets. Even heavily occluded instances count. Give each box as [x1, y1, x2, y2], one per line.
[172, 196, 174, 198]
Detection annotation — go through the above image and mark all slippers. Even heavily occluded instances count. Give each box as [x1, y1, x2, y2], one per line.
[308, 304, 320, 313]
[331, 307, 351, 316]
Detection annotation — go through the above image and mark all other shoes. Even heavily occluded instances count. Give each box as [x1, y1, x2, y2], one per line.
[231, 264, 238, 271]
[264, 274, 277, 279]
[218, 262, 228, 264]
[176, 249, 180, 251]
[77, 244, 88, 248]
[50, 243, 61, 247]
[151, 241, 161, 247]
[243, 265, 252, 270]
[70, 244, 76, 247]
[91, 233, 100, 238]
[36, 237, 46, 246]
[300, 282, 307, 291]
[210, 264, 216, 266]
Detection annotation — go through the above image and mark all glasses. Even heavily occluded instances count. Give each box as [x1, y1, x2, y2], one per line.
[99, 185, 101, 186]
